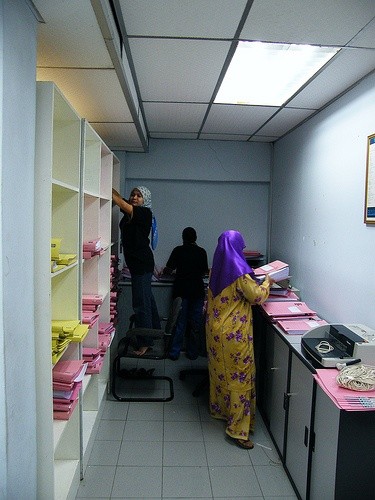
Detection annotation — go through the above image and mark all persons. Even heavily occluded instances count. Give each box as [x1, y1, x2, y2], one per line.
[158, 227, 209, 360]
[205, 230, 274, 449]
[111, 185, 161, 355]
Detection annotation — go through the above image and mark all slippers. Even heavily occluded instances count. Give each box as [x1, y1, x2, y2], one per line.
[227, 434, 254, 449]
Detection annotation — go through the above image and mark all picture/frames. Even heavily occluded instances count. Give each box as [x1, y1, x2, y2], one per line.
[362, 132, 375, 225]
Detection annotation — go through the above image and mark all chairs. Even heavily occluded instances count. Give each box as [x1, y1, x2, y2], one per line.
[110, 296, 185, 403]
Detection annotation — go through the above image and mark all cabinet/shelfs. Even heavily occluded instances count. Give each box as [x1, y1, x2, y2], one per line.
[120, 282, 375, 500]
[36, 69, 118, 500]
